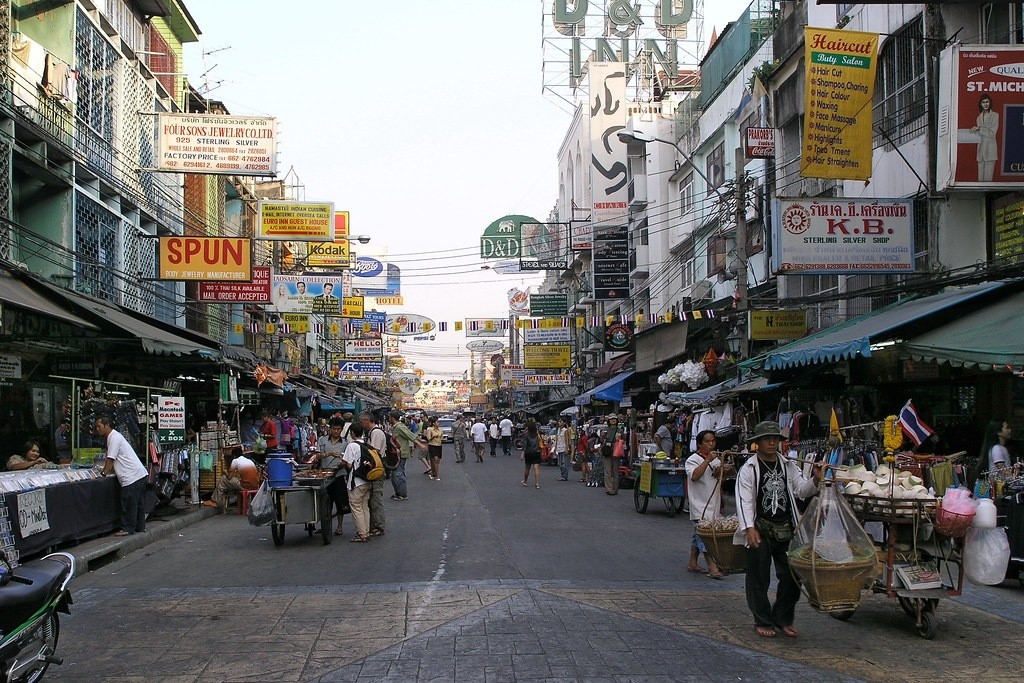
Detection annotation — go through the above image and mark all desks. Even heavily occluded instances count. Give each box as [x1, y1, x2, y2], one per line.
[0, 476, 122, 567]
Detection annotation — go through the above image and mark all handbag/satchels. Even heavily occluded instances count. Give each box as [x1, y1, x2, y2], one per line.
[199, 451, 213, 471]
[538, 432, 545, 450]
[601, 445, 612, 456]
[612, 439, 625, 457]
[247, 479, 276, 527]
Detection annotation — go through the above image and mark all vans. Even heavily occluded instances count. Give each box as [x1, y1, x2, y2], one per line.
[461, 411, 477, 428]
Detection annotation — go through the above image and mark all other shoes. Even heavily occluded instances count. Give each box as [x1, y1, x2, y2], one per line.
[391, 493, 408, 501]
[424, 467, 440, 482]
[203, 500, 217, 507]
[457, 450, 512, 464]
[558, 477, 567, 481]
[578, 478, 587, 482]
[115, 529, 136, 536]
[606, 491, 616, 495]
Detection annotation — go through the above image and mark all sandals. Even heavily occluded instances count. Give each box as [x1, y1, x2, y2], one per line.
[315, 526, 384, 542]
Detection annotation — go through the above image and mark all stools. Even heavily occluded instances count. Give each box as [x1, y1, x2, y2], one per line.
[238, 489, 260, 516]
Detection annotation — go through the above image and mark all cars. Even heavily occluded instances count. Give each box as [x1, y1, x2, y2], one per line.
[437, 419, 456, 443]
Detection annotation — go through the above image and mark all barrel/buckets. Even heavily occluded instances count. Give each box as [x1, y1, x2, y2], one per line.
[651, 457, 671, 468]
[266, 450, 293, 487]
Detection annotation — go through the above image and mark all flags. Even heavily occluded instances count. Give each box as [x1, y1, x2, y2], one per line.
[895, 401, 934, 447]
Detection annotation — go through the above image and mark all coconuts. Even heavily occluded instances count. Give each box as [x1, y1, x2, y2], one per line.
[836, 463, 935, 519]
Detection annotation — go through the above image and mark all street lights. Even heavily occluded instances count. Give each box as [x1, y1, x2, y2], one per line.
[616, 129, 749, 360]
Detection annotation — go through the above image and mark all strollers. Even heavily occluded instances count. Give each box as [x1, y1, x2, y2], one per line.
[586, 446, 604, 487]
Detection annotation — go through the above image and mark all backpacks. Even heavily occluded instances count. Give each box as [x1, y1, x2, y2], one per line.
[351, 440, 385, 481]
[370, 427, 401, 471]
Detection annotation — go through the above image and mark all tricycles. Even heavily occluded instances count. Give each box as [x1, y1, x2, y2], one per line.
[270, 459, 337, 546]
[632, 446, 687, 515]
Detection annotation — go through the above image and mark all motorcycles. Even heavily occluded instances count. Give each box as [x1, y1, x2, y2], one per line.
[0, 548, 77, 683]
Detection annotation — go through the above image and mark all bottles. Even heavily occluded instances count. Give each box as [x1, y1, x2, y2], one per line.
[675, 457, 679, 468]
[972, 498, 997, 529]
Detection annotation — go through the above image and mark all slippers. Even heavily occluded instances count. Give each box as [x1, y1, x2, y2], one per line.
[688, 567, 708, 575]
[521, 480, 528, 486]
[752, 626, 775, 637]
[535, 486, 539, 489]
[706, 572, 724, 578]
[776, 625, 799, 637]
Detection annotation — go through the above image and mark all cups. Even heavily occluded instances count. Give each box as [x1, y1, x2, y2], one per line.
[292, 481, 299, 487]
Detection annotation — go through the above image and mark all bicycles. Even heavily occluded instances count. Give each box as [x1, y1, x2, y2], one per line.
[513, 435, 526, 450]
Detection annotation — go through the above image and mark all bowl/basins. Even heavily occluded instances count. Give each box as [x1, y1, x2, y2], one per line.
[320, 468, 333, 472]
[295, 477, 326, 486]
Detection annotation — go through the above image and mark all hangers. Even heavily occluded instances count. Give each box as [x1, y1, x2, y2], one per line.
[789, 435, 880, 461]
[698, 394, 760, 415]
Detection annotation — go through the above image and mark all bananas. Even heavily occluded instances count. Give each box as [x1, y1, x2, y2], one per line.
[656, 451, 666, 459]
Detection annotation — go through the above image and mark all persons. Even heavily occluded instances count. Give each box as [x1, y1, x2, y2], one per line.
[685, 430, 725, 580]
[96, 416, 150, 537]
[734, 420, 830, 637]
[55, 420, 72, 464]
[202, 408, 677, 542]
[6, 440, 49, 471]
[979, 418, 1013, 476]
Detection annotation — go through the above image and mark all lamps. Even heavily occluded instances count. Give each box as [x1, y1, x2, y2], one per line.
[339, 379, 358, 403]
[727, 328, 750, 356]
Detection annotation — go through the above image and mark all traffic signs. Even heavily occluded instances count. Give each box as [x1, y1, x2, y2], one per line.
[258, 200, 336, 242]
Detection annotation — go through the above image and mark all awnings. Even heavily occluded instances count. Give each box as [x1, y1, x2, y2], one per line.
[574, 371, 636, 405]
[0, 274, 254, 362]
[714, 281, 1024, 396]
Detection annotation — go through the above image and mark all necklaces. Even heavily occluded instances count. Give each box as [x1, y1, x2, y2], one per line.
[758, 455, 778, 479]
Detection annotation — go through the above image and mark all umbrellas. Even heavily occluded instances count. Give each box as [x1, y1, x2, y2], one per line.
[559, 406, 592, 420]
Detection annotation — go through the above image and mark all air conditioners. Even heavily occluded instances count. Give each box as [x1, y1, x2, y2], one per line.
[691, 279, 712, 302]
[12, 260, 29, 272]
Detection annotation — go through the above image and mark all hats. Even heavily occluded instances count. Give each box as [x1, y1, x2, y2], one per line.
[608, 413, 618, 420]
[745, 421, 788, 441]
